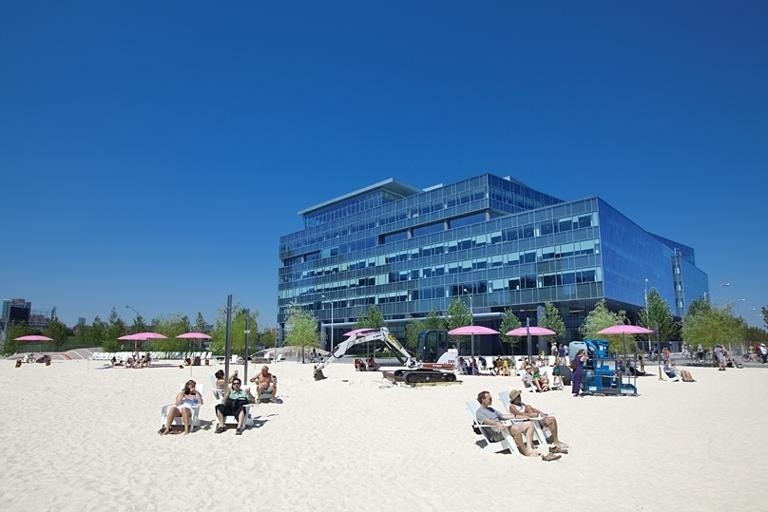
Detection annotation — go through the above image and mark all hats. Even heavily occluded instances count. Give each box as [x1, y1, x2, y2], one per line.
[509, 389, 522, 402]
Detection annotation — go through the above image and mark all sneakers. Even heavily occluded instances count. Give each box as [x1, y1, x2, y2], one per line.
[215, 426, 227, 433]
[541, 446, 567, 462]
[234, 428, 243, 435]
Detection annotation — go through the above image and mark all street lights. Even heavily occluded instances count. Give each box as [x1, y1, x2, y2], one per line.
[124, 304, 142, 351]
[642, 277, 651, 352]
[702, 279, 763, 361]
[462, 287, 476, 377]
[318, 295, 337, 355]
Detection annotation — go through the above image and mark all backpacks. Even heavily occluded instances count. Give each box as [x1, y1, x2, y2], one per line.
[570, 360, 577, 370]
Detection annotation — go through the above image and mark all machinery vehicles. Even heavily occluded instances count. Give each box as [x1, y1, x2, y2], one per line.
[312, 324, 464, 391]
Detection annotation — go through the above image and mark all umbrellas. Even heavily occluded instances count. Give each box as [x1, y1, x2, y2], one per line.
[597, 323, 653, 363]
[505, 326, 557, 367]
[135, 331, 168, 360]
[446, 326, 500, 376]
[115, 334, 148, 362]
[175, 331, 212, 362]
[11, 335, 55, 364]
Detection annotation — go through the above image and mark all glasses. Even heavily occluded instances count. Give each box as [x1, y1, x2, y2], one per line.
[233, 383, 240, 384]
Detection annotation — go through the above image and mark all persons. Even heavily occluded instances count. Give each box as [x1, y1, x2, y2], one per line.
[213, 370, 239, 395]
[214, 379, 255, 435]
[355, 358, 366, 371]
[158, 379, 203, 435]
[474, 389, 542, 458]
[366, 354, 379, 371]
[507, 387, 570, 449]
[250, 365, 279, 404]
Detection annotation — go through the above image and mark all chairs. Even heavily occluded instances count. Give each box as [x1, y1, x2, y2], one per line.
[458, 354, 551, 378]
[617, 353, 644, 376]
[465, 398, 527, 456]
[522, 362, 574, 393]
[663, 367, 684, 385]
[354, 355, 382, 372]
[158, 371, 275, 429]
[498, 390, 551, 447]
